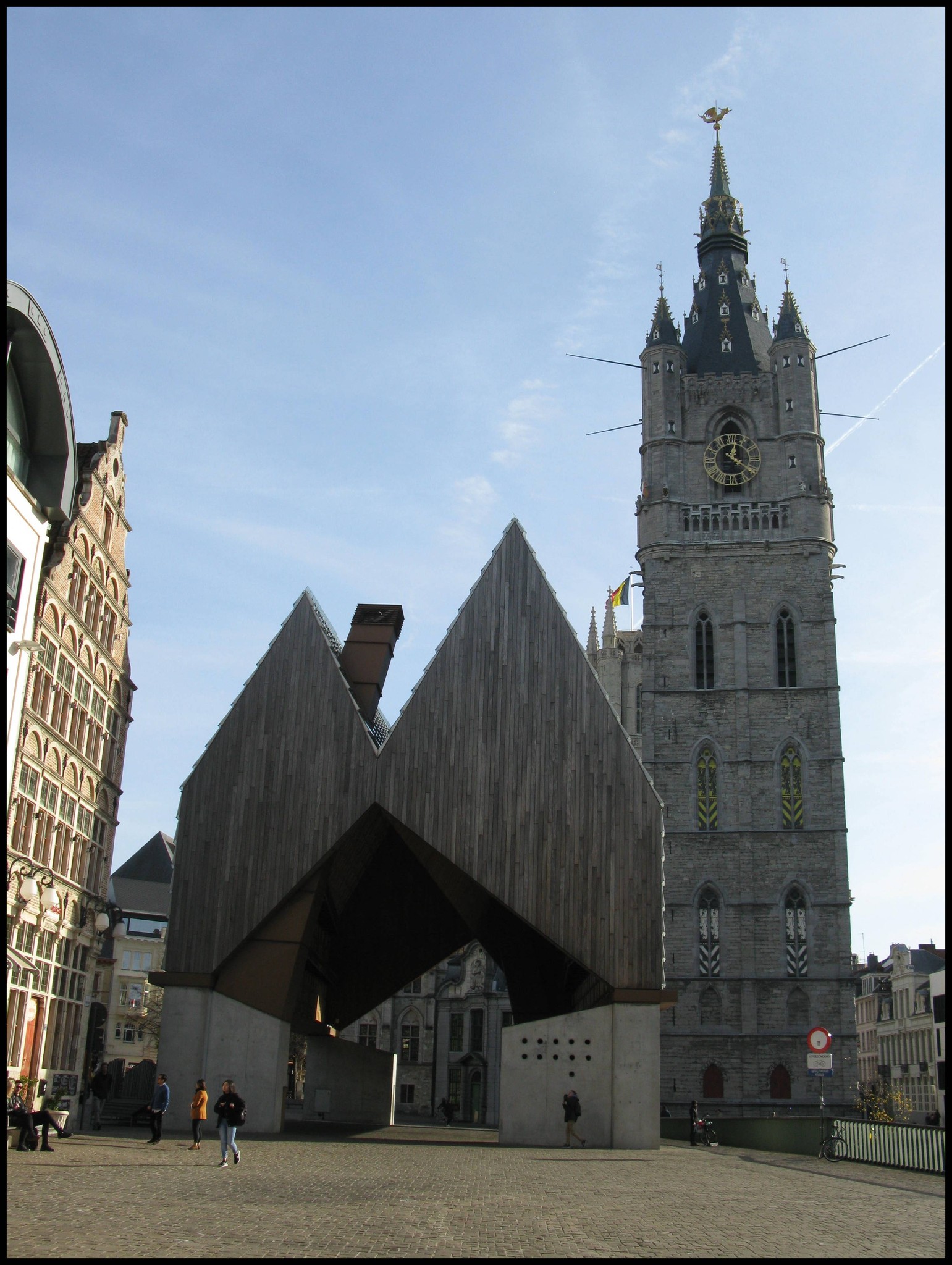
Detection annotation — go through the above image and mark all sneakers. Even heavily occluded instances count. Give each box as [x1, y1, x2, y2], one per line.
[218, 1160, 228, 1167]
[233, 1151, 240, 1164]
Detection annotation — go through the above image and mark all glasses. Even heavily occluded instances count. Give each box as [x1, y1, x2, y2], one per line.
[16, 1085, 23, 1089]
[569, 1092, 573, 1093]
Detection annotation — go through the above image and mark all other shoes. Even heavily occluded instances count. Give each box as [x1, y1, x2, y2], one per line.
[57, 1130, 73, 1138]
[562, 1143, 570, 1148]
[147, 1136, 160, 1144]
[25, 1132, 40, 1142]
[188, 1145, 198, 1150]
[690, 1143, 699, 1147]
[94, 1123, 101, 1130]
[198, 1144, 200, 1150]
[16, 1144, 30, 1152]
[40, 1143, 54, 1152]
[581, 1139, 587, 1148]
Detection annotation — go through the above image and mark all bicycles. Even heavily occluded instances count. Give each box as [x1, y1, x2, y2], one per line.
[698, 1113, 716, 1147]
[432, 1108, 458, 1126]
[819, 1120, 848, 1162]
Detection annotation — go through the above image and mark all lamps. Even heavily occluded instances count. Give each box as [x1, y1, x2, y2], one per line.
[80, 896, 110, 933]
[7, 857, 37, 903]
[24, 867, 59, 909]
[101, 905, 127, 942]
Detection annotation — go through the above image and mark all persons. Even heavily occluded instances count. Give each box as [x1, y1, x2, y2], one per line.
[438, 1097, 454, 1127]
[147, 1074, 170, 1145]
[7, 1077, 73, 1152]
[91, 1062, 111, 1130]
[214, 1080, 247, 1168]
[925, 1109, 941, 1127]
[689, 1099, 699, 1146]
[190, 1078, 208, 1150]
[660, 1105, 671, 1117]
[563, 1090, 587, 1148]
[767, 1110, 775, 1116]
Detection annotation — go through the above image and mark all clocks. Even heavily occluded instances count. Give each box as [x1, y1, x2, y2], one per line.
[703, 433, 761, 485]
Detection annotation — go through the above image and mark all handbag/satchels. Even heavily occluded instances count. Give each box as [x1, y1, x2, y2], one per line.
[229, 1103, 247, 1126]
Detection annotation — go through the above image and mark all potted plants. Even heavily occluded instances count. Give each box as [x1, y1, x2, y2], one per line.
[45, 1086, 70, 1129]
